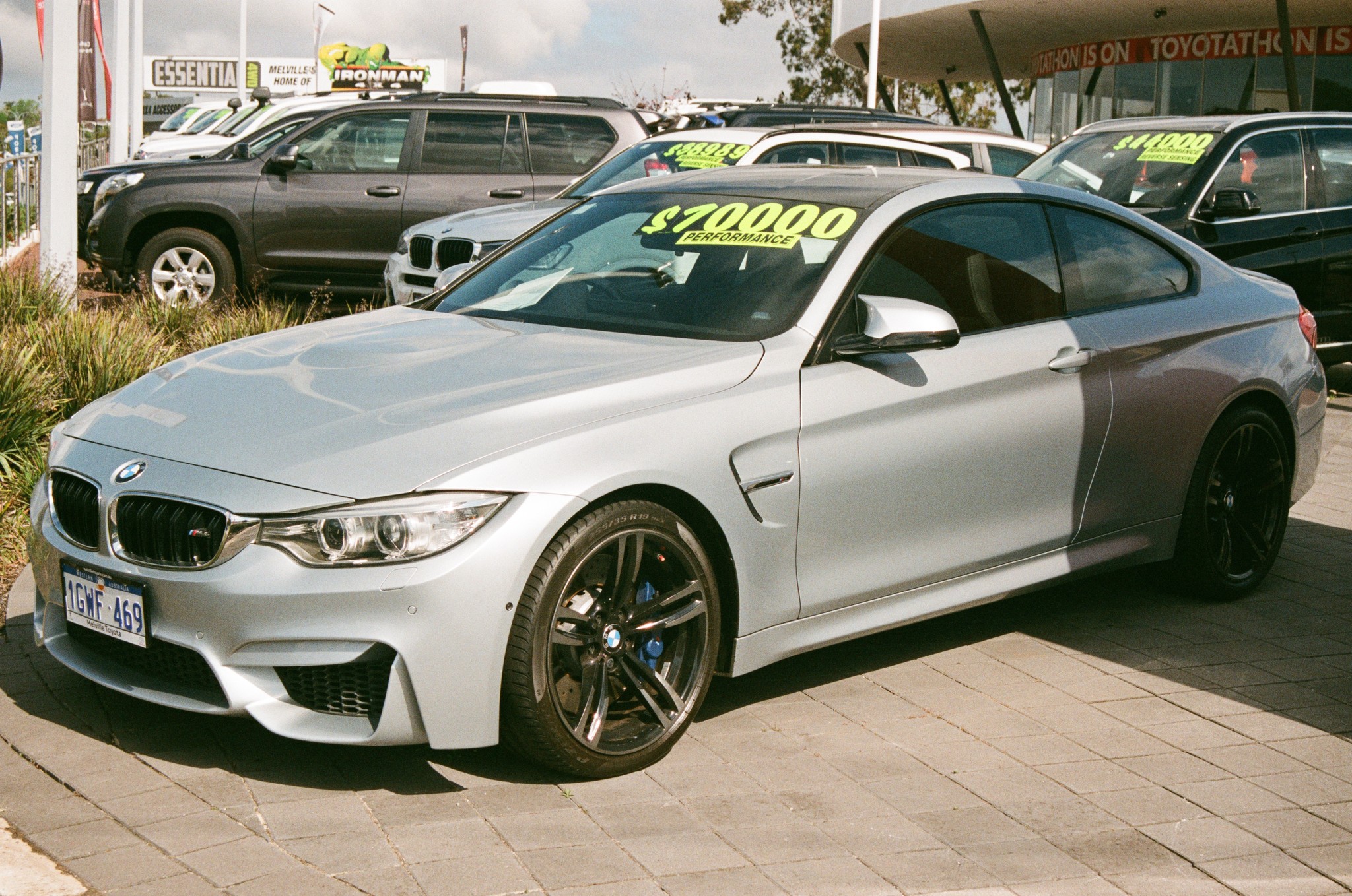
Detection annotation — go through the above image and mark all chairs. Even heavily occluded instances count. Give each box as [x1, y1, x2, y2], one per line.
[704, 239, 810, 338]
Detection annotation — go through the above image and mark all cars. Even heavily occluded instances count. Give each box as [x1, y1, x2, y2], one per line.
[27, 164, 1327, 781]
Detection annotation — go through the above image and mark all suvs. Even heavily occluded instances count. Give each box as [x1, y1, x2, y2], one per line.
[75, 87, 405, 290]
[1015, 109, 1352, 371]
[384, 123, 973, 305]
[659, 95, 1259, 206]
[86, 94, 678, 315]
